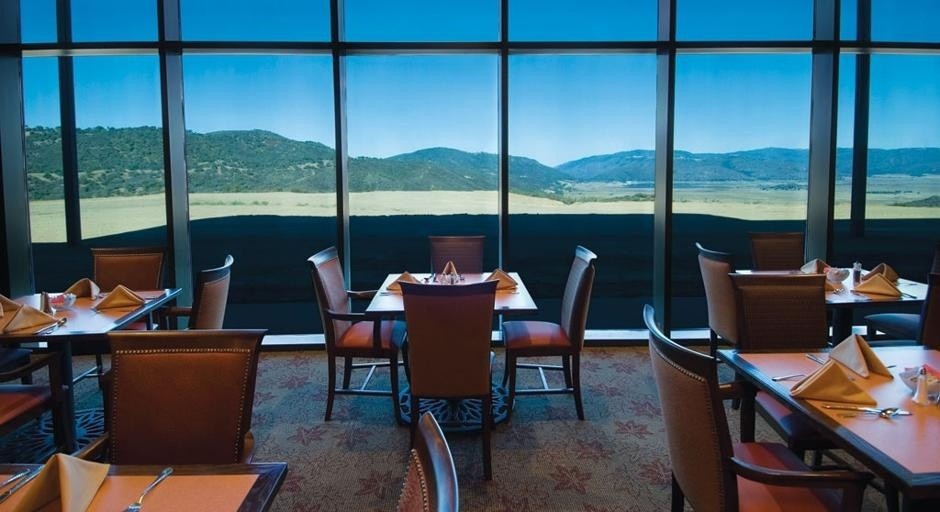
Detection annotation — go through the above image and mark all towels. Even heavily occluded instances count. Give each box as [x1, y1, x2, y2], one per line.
[851, 274, 902, 297]
[862, 263, 900, 281]
[831, 333, 894, 379]
[14, 452, 110, 510]
[3, 304, 59, 332]
[825, 278, 836, 291]
[802, 260, 829, 274]
[95, 283, 144, 311]
[0, 294, 22, 317]
[64, 278, 100, 300]
[387, 271, 421, 290]
[442, 260, 458, 279]
[484, 269, 517, 289]
[790, 360, 878, 407]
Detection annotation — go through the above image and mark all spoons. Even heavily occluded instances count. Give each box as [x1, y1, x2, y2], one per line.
[47, 317, 67, 334]
[834, 407, 899, 417]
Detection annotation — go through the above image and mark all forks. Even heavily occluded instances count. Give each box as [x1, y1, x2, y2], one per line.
[123, 465, 174, 512]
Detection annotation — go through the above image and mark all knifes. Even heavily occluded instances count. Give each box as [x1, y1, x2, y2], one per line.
[822, 403, 911, 416]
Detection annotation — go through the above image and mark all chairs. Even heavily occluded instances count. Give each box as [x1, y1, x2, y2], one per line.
[920, 273, 940, 347]
[171, 254, 235, 328]
[0, 384, 70, 454]
[427, 235, 486, 273]
[89, 246, 167, 331]
[501, 245, 598, 425]
[307, 245, 409, 425]
[642, 303, 875, 512]
[397, 280, 499, 481]
[862, 242, 940, 341]
[695, 241, 740, 410]
[748, 232, 803, 272]
[69, 328, 269, 466]
[729, 273, 842, 460]
[393, 410, 460, 512]
[0, 343, 50, 384]
